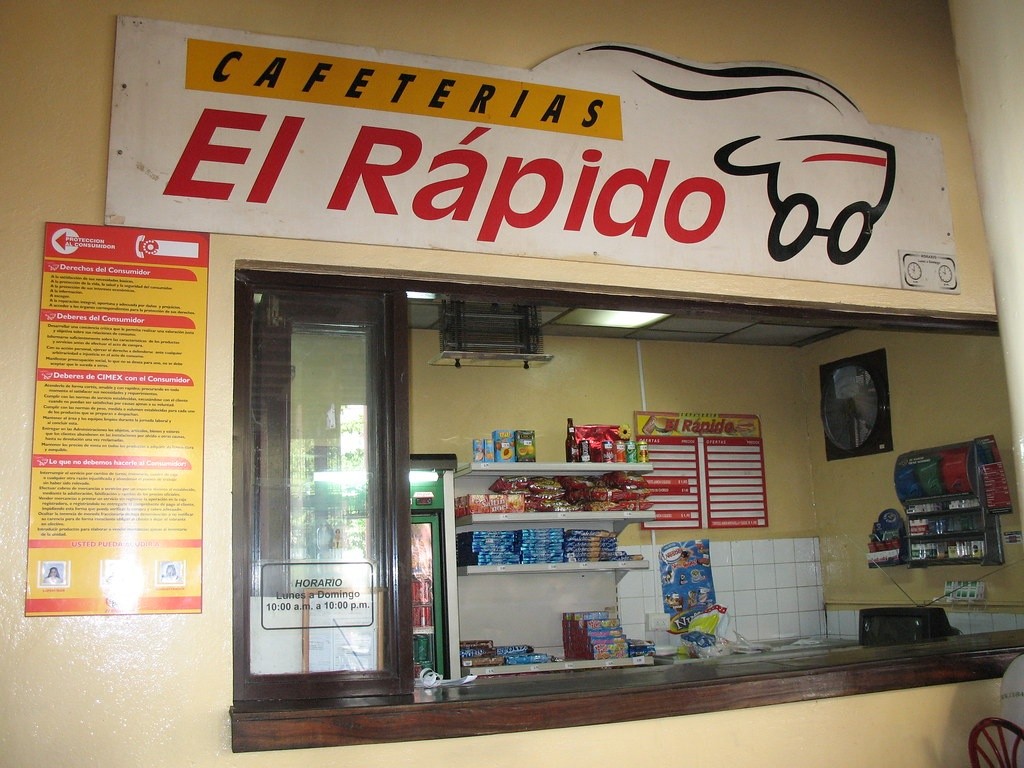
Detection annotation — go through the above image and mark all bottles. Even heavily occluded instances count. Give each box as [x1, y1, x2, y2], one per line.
[565, 418, 578, 462]
[643, 416, 657, 436]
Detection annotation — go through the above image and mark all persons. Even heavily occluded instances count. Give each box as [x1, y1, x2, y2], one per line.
[162, 565, 182, 584]
[44, 567, 64, 583]
[333, 530, 344, 548]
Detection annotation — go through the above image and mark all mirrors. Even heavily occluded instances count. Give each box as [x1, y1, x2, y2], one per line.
[818, 347, 894, 462]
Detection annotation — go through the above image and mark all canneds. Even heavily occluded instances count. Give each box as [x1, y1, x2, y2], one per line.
[579, 440, 591, 462]
[412, 578, 432, 678]
[601, 440, 649, 463]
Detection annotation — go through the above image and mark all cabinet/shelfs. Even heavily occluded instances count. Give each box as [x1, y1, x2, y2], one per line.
[906, 492, 1005, 567]
[453, 418, 655, 677]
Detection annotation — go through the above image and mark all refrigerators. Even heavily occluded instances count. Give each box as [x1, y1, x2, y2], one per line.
[409, 453, 460, 681]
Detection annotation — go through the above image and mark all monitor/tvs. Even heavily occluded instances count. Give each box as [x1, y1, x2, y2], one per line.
[859, 606, 960, 646]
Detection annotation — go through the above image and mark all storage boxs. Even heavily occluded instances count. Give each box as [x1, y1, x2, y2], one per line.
[514, 430, 537, 463]
[488, 494, 507, 513]
[506, 493, 525, 513]
[466, 494, 489, 515]
[492, 430, 516, 463]
